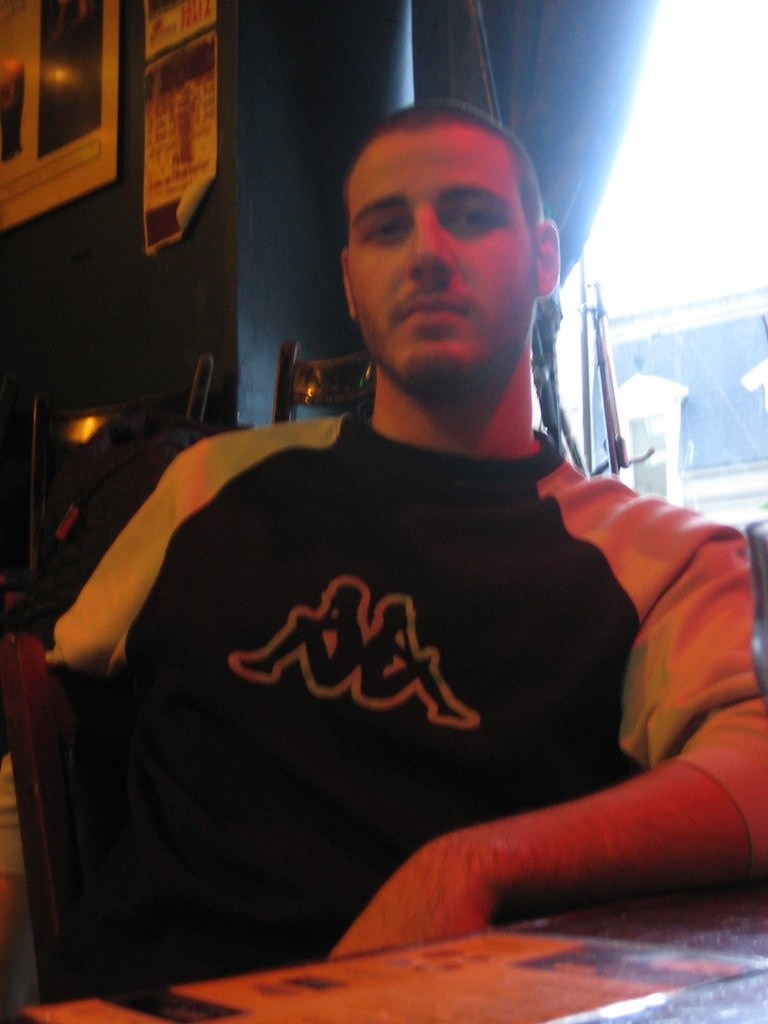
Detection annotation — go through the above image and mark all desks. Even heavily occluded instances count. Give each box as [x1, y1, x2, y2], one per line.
[19, 879, 768, 1024]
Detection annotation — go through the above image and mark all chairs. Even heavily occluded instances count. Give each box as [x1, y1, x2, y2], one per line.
[27, 352, 214, 571]
[0, 631, 136, 1002]
[271, 340, 375, 424]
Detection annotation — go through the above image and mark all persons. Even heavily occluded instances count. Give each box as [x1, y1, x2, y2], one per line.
[0, 97, 768, 1024]
